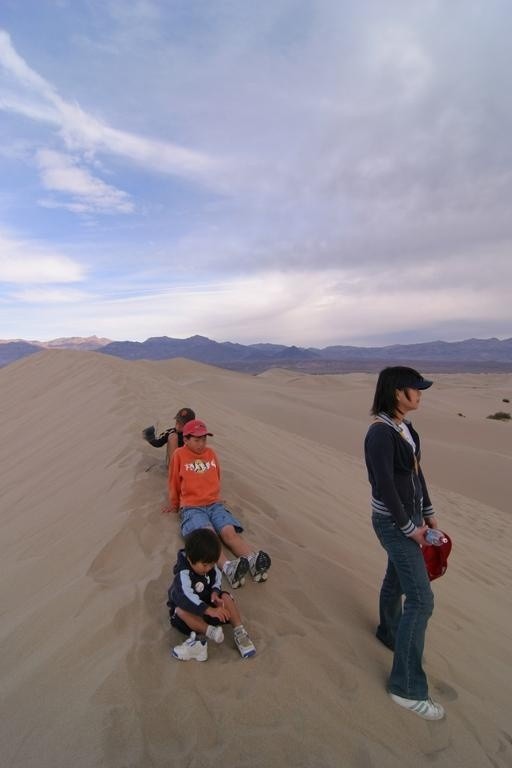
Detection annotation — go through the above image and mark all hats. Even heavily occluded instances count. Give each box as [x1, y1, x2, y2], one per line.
[183, 419, 213, 438]
[173, 408, 195, 424]
[396, 372, 433, 390]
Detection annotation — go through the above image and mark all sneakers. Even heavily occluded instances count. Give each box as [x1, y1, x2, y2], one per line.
[234, 626, 256, 659]
[171, 630, 208, 662]
[248, 551, 272, 583]
[224, 556, 250, 589]
[389, 691, 444, 720]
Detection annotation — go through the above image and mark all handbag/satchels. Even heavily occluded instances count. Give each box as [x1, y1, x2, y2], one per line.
[417, 527, 452, 580]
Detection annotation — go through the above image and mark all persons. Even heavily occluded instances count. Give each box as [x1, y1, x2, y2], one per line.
[363, 365, 450, 720]
[160, 419, 271, 588]
[165, 528, 255, 660]
[143, 405, 194, 461]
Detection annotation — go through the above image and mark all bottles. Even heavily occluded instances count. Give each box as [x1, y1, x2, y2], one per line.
[424, 527, 447, 546]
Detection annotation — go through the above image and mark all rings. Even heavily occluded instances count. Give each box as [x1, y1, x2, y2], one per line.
[419, 543, 423, 548]
